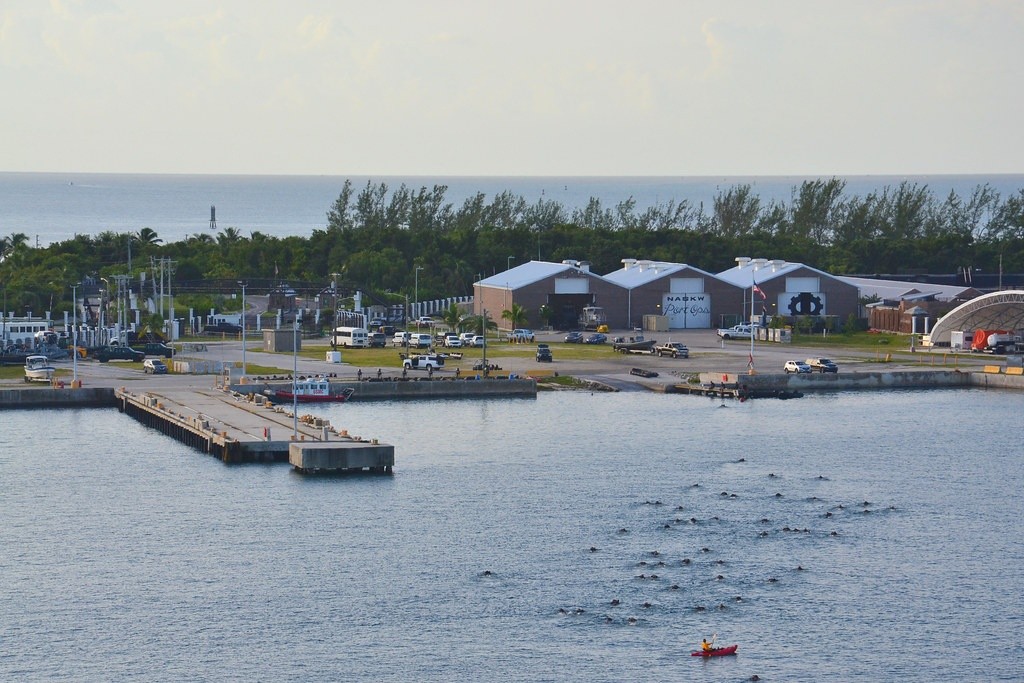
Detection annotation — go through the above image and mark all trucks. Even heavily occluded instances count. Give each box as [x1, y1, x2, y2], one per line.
[367, 316, 474, 348]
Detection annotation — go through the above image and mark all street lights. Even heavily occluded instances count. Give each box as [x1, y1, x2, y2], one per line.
[292, 319, 305, 440]
[415, 266, 426, 320]
[70, 281, 85, 387]
[507, 256, 515, 284]
[100, 278, 111, 329]
[237, 279, 249, 384]
[734, 256, 786, 375]
[332, 273, 340, 351]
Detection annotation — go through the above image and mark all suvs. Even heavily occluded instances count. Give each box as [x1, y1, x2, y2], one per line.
[402, 354, 446, 372]
[782, 357, 839, 377]
[536, 348, 554, 362]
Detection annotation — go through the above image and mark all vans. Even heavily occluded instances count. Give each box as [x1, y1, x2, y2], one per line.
[329, 327, 369, 348]
[109, 330, 177, 358]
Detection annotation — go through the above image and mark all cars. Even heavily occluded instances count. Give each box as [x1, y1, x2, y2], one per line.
[585, 333, 608, 344]
[564, 332, 584, 344]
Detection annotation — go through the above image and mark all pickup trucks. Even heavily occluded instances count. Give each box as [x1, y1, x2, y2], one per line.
[506, 328, 537, 343]
[470, 336, 489, 347]
[417, 316, 435, 326]
[94, 346, 145, 363]
[655, 342, 689, 359]
[204, 322, 243, 333]
[718, 323, 761, 341]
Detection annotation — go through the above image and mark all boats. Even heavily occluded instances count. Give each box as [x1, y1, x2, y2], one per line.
[264, 378, 357, 404]
[24, 356, 56, 385]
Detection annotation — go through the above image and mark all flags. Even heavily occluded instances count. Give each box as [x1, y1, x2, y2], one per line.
[753, 281, 766, 299]
